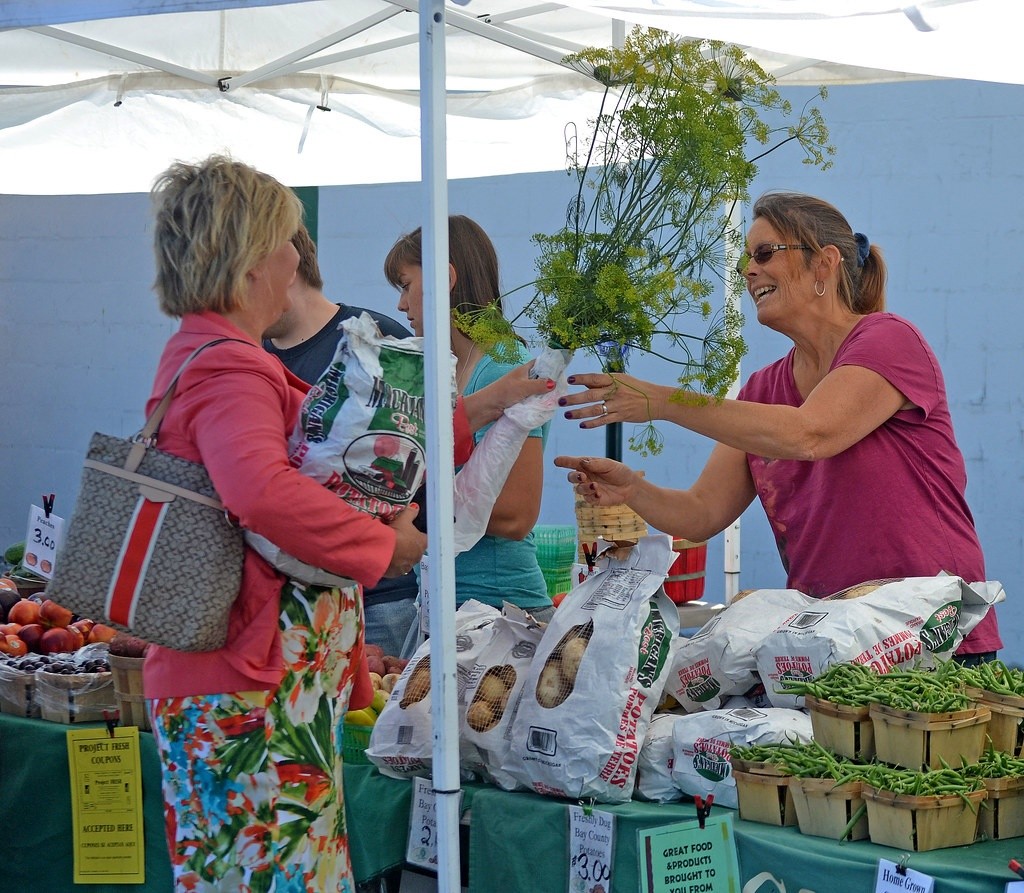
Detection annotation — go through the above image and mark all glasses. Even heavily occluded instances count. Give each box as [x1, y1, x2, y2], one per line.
[736, 244, 811, 275]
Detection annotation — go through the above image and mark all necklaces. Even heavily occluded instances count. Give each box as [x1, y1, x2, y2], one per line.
[457, 341, 476, 385]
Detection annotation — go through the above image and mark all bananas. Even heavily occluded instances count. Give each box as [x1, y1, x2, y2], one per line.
[344, 688, 386, 727]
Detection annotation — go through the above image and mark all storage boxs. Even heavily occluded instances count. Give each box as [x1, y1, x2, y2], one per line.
[981, 776, 1024, 840]
[34, 666, 120, 725]
[860, 779, 988, 850]
[964, 685, 1024, 758]
[0, 657, 38, 718]
[533, 524, 577, 599]
[730, 756, 798, 827]
[789, 775, 866, 841]
[342, 722, 376, 764]
[804, 694, 874, 760]
[868, 702, 990, 773]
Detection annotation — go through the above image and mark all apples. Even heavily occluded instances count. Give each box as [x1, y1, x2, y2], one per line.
[0, 577, 156, 658]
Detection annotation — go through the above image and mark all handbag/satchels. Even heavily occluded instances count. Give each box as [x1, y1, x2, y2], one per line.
[45, 338, 263, 650]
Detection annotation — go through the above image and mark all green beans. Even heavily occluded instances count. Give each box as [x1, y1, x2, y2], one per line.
[726, 658, 1024, 846]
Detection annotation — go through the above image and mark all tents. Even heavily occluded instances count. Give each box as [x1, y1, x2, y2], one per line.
[0, 0, 1024, 893]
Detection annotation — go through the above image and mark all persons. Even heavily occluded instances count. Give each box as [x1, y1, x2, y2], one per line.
[555, 189, 1004, 668]
[143, 150, 554, 893]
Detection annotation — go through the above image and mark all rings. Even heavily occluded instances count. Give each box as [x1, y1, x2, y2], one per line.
[602, 404, 608, 415]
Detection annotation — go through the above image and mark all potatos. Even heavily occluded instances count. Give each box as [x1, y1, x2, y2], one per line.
[363, 627, 590, 733]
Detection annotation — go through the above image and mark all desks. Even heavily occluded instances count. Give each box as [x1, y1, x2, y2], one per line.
[0, 713, 174, 893]
[342, 762, 740, 893]
[733, 820, 1024, 893]
